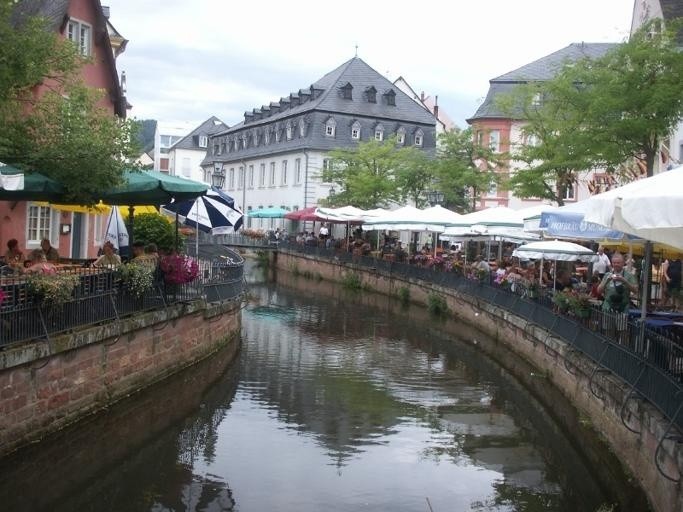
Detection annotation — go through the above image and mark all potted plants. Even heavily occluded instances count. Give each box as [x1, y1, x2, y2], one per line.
[554, 289, 592, 319]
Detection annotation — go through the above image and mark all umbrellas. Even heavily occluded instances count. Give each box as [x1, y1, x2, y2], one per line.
[0, 158, 244, 263]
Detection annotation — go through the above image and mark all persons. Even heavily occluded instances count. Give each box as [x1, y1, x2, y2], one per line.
[0, 237, 124, 280]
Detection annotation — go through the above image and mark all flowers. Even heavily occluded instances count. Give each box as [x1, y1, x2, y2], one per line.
[0, 252, 201, 319]
